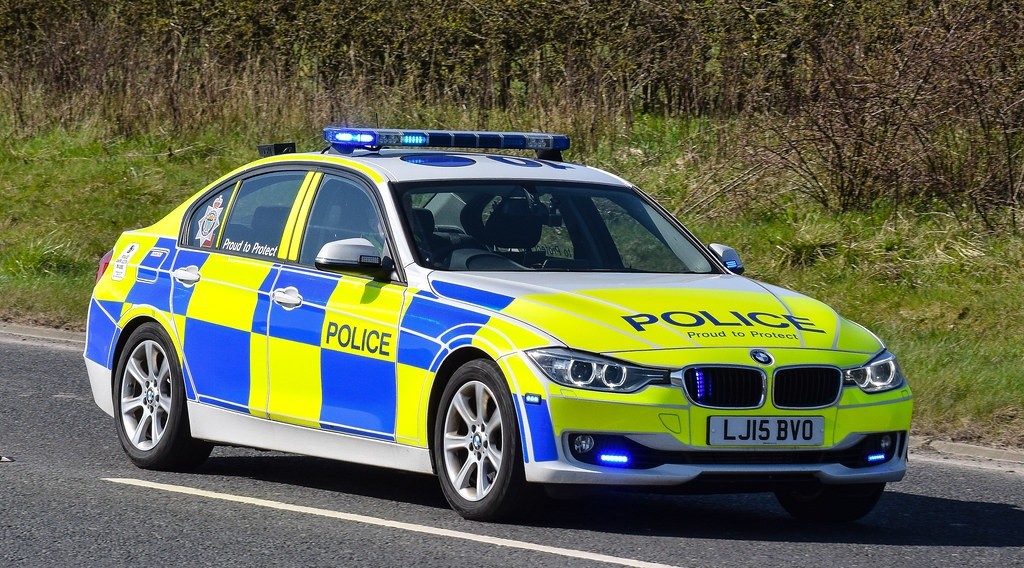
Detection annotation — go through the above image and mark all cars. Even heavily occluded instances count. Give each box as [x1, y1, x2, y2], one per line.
[82, 130, 912, 522]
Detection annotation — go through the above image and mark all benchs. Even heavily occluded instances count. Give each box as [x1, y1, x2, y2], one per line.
[225, 205, 488, 253]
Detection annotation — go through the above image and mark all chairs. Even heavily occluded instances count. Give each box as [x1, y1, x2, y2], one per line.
[318, 200, 453, 270]
[466, 197, 604, 273]
